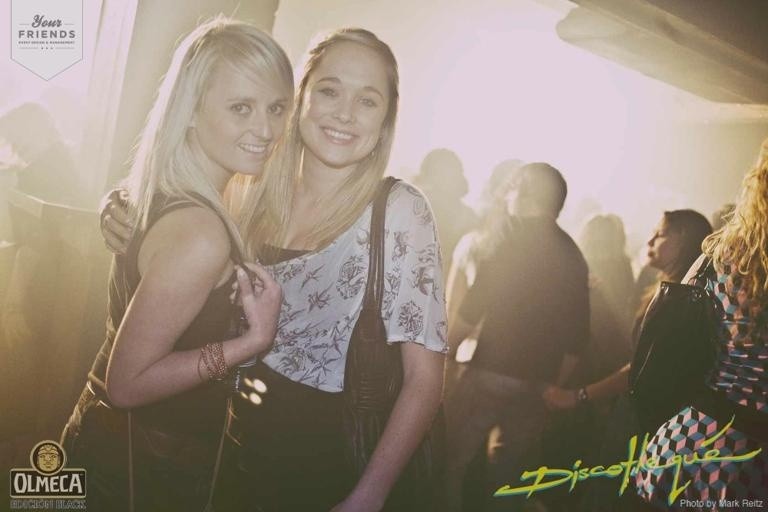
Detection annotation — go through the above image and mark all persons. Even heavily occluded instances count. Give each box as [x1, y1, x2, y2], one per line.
[96, 26, 448, 512]
[539, 209, 735, 439]
[633, 141, 767, 512]
[406, 147, 471, 269]
[443, 163, 520, 347]
[580, 212, 637, 310]
[443, 161, 592, 512]
[46, 18, 284, 511]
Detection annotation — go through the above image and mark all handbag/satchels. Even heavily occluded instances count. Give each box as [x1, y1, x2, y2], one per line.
[340, 171, 453, 495]
[623, 278, 729, 406]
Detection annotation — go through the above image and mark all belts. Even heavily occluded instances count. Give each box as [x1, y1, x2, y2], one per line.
[71, 377, 223, 478]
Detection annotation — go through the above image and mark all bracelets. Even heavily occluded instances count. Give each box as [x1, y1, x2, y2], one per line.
[575, 384, 587, 407]
[198, 341, 230, 386]
